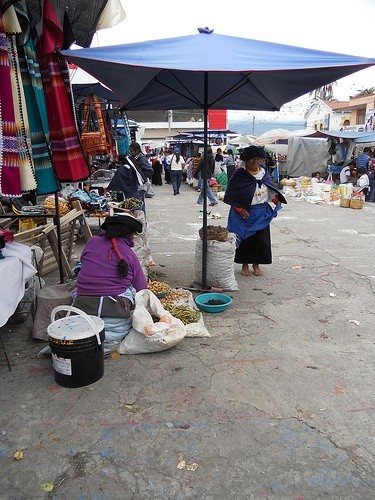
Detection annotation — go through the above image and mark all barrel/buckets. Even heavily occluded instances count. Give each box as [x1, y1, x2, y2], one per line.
[46, 305, 105, 388]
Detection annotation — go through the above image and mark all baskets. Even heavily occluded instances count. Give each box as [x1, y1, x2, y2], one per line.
[199, 225, 229, 242]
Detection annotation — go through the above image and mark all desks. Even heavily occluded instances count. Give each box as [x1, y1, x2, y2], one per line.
[61, 212, 111, 232]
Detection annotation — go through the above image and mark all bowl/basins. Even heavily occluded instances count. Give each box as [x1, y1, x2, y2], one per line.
[155, 286, 170, 298]
[196, 292, 232, 312]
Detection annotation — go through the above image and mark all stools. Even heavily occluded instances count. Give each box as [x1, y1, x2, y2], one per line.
[213, 185, 222, 191]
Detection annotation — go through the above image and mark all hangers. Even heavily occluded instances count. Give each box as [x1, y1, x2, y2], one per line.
[77, 87, 107, 104]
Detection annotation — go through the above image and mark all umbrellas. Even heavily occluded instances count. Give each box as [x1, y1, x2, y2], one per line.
[59, 27, 375, 288]
[72, 82, 121, 119]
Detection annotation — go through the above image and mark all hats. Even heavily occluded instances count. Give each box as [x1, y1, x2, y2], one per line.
[174, 148, 180, 154]
[240, 145, 266, 162]
[100, 215, 143, 234]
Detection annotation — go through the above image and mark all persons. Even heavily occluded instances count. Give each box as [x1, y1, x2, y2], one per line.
[339, 147, 375, 198]
[107, 143, 154, 212]
[144, 151, 173, 184]
[313, 172, 325, 182]
[222, 146, 287, 276]
[74, 213, 146, 319]
[168, 147, 185, 195]
[215, 148, 223, 162]
[221, 149, 235, 181]
[193, 147, 218, 206]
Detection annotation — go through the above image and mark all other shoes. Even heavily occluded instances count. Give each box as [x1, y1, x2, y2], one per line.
[209, 202, 218, 206]
[197, 201, 202, 204]
[173, 193, 176, 195]
[177, 192, 179, 194]
[196, 189, 200, 192]
